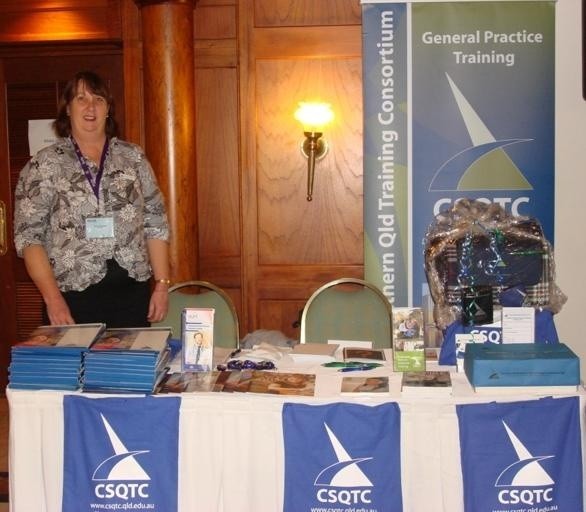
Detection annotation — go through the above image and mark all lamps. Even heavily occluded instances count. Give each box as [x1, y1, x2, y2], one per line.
[289, 95, 338, 202]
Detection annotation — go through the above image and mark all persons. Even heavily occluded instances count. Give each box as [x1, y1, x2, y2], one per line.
[12, 70, 173, 328]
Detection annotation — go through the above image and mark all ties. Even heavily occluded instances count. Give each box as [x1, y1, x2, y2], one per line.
[196, 346, 200, 364]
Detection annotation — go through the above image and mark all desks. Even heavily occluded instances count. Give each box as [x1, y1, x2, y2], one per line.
[3, 343, 586, 511]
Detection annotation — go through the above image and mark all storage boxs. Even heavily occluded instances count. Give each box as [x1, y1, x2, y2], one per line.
[462, 339, 580, 387]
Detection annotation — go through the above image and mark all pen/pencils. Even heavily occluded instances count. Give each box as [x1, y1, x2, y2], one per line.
[320, 362, 383, 368]
[337, 367, 373, 372]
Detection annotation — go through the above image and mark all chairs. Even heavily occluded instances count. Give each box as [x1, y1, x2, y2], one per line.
[149, 273, 242, 352]
[295, 275, 396, 354]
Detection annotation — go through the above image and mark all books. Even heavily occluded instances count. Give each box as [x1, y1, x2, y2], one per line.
[502, 306, 537, 343]
[341, 376, 389, 392]
[7, 322, 174, 396]
[343, 345, 386, 364]
[289, 342, 340, 361]
[156, 370, 316, 397]
[402, 371, 452, 394]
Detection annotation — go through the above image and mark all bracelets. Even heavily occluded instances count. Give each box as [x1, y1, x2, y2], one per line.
[154, 278, 171, 285]
